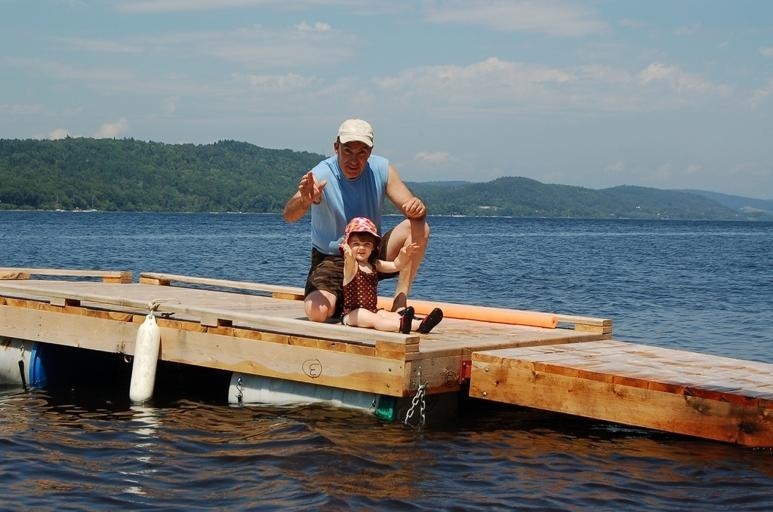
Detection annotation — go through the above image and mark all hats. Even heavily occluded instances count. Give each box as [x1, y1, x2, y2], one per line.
[338, 119, 374, 148]
[339, 217, 384, 259]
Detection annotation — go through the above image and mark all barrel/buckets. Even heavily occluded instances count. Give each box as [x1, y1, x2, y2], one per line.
[0, 335, 57, 388]
[227, 371, 407, 424]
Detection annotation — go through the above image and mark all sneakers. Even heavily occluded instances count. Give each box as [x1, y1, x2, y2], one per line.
[400, 307, 414, 334]
[419, 308, 443, 333]
[398, 309, 423, 320]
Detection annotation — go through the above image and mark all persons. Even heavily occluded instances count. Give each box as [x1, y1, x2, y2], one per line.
[281, 117, 431, 326]
[334, 216, 443, 336]
